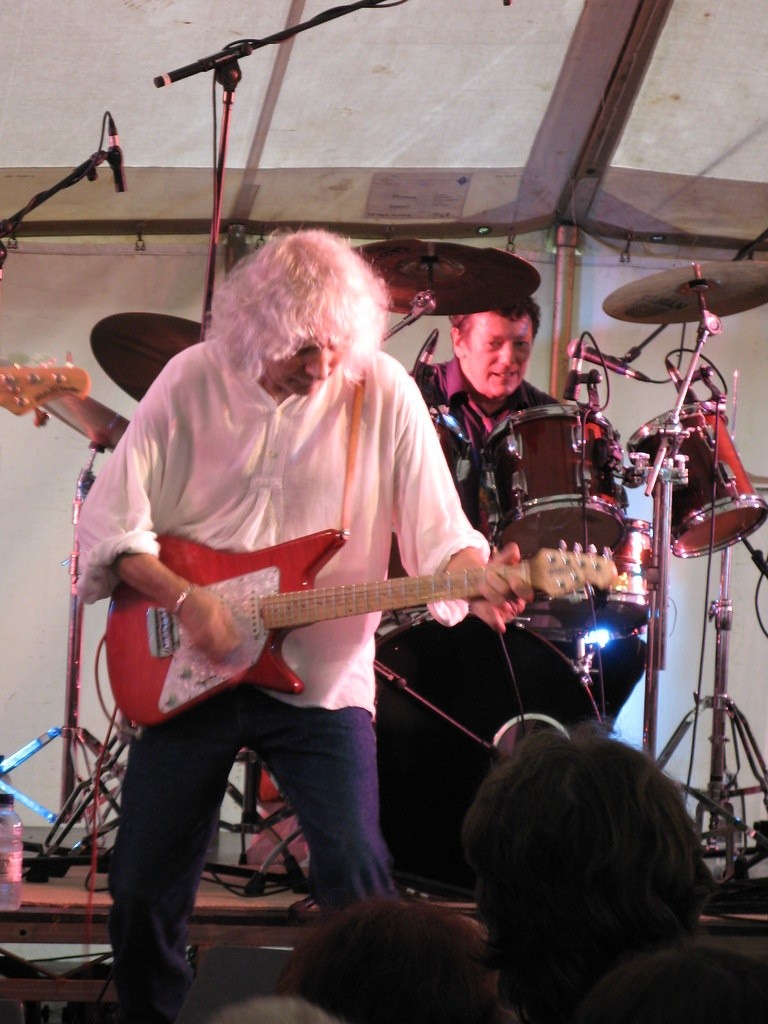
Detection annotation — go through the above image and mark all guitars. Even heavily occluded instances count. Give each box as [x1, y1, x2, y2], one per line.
[0, 351, 93, 426]
[104, 527, 620, 734]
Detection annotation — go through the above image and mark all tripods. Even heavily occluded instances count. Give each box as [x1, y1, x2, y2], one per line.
[1, 442, 126, 823]
[653, 546, 768, 886]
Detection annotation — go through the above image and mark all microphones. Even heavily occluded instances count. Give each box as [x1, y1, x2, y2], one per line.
[412, 336, 439, 380]
[567, 339, 650, 382]
[108, 117, 127, 192]
[563, 337, 585, 401]
[665, 359, 699, 404]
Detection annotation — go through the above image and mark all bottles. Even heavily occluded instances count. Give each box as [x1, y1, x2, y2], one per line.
[0, 756, 12, 786]
[0, 793, 21, 910]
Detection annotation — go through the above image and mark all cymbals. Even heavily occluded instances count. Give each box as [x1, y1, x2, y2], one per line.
[89, 311, 202, 405]
[40, 393, 131, 454]
[601, 259, 768, 326]
[350, 234, 543, 318]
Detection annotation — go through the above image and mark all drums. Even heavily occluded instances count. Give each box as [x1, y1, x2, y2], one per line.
[429, 411, 482, 531]
[375, 611, 604, 901]
[625, 401, 768, 562]
[479, 404, 629, 562]
[523, 515, 654, 643]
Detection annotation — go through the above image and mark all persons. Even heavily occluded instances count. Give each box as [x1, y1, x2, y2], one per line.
[79, 227, 533, 1024]
[217, 890, 516, 1024]
[447, 727, 768, 1023]
[407, 288, 630, 633]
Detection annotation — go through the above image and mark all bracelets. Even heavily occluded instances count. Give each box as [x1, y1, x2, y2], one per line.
[174, 583, 196, 615]
